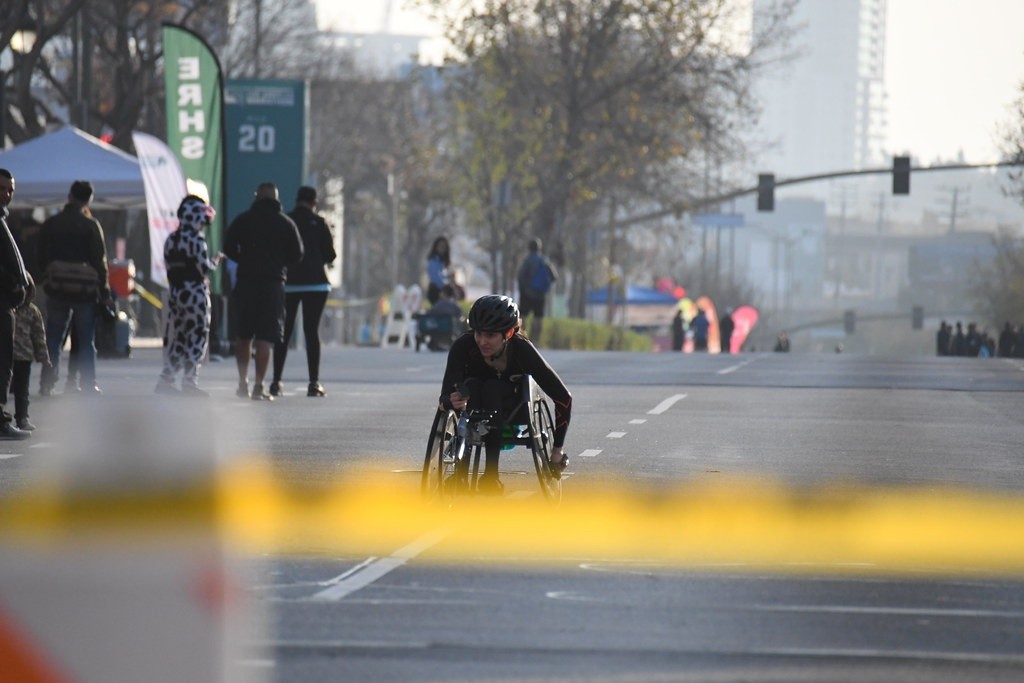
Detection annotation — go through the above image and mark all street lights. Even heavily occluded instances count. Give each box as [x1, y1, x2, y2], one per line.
[713, 138, 774, 322]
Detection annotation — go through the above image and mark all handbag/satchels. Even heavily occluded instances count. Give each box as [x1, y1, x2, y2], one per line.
[42, 259, 100, 303]
[95, 303, 129, 360]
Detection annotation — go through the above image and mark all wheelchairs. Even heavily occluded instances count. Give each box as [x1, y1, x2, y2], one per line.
[419, 385, 562, 510]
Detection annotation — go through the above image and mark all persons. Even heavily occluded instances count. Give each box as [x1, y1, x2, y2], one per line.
[269, 185, 336, 398]
[223, 183, 305, 402]
[428, 236, 454, 351]
[749, 330, 844, 353]
[439, 294, 572, 495]
[153, 195, 224, 397]
[720, 312, 734, 353]
[9, 270, 53, 430]
[936, 319, 1024, 358]
[27, 180, 111, 398]
[673, 310, 683, 350]
[689, 309, 709, 352]
[0, 167, 31, 440]
[518, 237, 559, 349]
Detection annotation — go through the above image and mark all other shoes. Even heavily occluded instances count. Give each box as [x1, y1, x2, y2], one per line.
[251, 384, 271, 400]
[39, 387, 55, 396]
[182, 384, 209, 398]
[64, 387, 81, 393]
[0, 419, 31, 441]
[308, 382, 326, 396]
[81, 385, 102, 395]
[16, 417, 36, 430]
[270, 382, 286, 395]
[153, 383, 181, 395]
[237, 383, 250, 398]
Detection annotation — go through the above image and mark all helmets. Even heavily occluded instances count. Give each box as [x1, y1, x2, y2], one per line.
[469, 295, 519, 333]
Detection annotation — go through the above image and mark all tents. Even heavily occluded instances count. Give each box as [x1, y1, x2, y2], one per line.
[0, 123, 148, 209]
